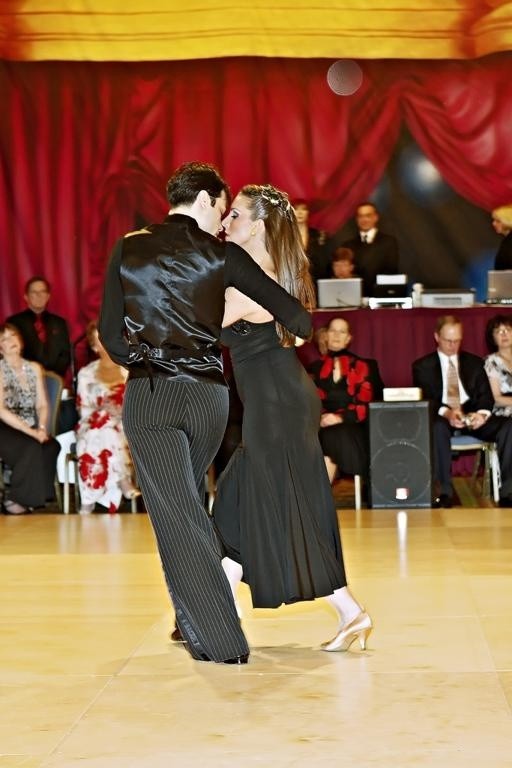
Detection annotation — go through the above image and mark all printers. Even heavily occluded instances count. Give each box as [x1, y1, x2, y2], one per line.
[368, 283, 412, 310]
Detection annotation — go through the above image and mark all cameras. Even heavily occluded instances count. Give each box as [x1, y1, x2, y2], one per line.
[458, 414, 474, 426]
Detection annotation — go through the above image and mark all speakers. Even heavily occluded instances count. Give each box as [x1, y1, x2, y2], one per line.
[369, 401, 433, 508]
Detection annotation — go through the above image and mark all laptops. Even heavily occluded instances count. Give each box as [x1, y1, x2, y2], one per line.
[317, 277, 362, 308]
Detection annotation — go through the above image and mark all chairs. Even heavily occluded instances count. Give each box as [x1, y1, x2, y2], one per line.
[64, 442, 138, 514]
[0, 369, 64, 510]
[450, 435, 490, 501]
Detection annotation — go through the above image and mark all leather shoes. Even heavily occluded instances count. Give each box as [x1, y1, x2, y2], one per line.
[432, 495, 450, 508]
[125, 488, 142, 499]
[199, 649, 249, 664]
[1, 500, 33, 515]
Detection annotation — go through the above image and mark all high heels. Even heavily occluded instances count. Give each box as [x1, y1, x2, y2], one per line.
[320, 610, 372, 651]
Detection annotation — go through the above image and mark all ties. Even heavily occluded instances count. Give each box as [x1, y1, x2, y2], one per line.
[447, 359, 460, 410]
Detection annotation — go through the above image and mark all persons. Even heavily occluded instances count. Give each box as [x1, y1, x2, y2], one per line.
[100, 163, 375, 667]
[289, 198, 400, 296]
[492, 206, 512, 271]
[305, 315, 511, 510]
[0, 276, 141, 515]
